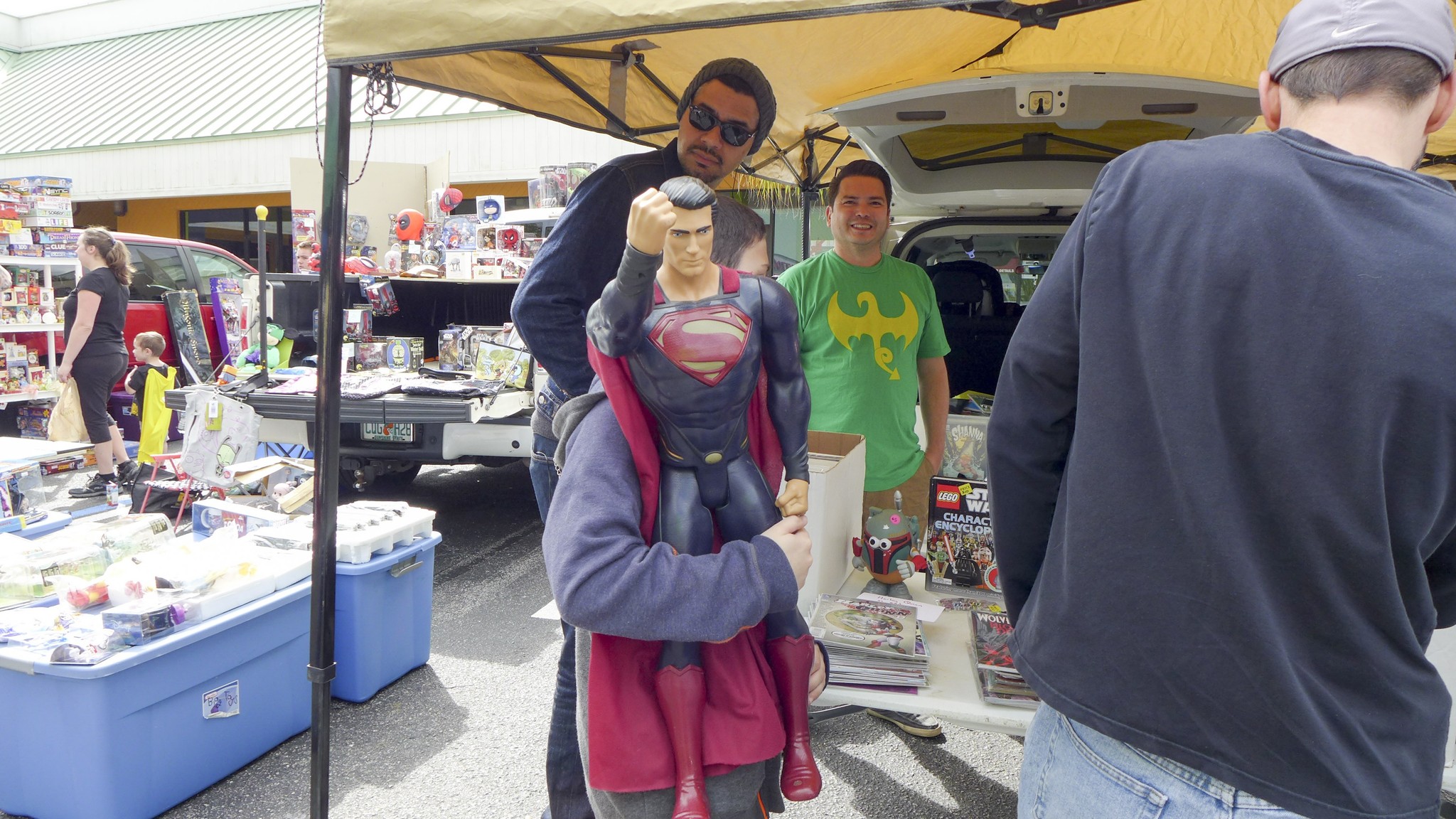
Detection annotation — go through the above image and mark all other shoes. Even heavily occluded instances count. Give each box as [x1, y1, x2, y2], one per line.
[160, 465, 167, 470]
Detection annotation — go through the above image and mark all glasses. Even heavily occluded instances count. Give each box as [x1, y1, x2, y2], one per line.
[687, 98, 758, 147]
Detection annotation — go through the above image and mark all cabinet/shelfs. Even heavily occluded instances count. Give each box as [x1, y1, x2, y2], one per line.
[0, 256, 83, 403]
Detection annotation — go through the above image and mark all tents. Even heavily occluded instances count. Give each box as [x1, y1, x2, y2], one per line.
[310, 0, 1456, 819]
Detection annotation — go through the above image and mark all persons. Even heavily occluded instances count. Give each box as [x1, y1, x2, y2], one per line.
[124, 331, 169, 470]
[985, 0, 1456, 819]
[540, 196, 770, 819]
[509, 55, 779, 819]
[777, 160, 950, 548]
[55, 226, 139, 497]
[586, 176, 823, 819]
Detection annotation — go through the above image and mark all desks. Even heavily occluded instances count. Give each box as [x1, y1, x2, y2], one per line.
[810, 567, 1036, 737]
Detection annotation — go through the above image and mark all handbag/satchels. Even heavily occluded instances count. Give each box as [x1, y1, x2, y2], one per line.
[47, 377, 89, 443]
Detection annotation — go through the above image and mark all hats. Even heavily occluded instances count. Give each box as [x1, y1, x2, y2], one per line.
[676, 58, 778, 156]
[1267, 0, 1456, 83]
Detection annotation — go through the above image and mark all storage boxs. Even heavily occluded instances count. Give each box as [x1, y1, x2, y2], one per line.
[109, 390, 183, 442]
[776, 430, 867, 618]
[101, 592, 201, 645]
[399, 163, 597, 279]
[330, 531, 443, 701]
[364, 275, 401, 317]
[209, 276, 243, 368]
[160, 288, 215, 384]
[0, 576, 314, 819]
[438, 322, 512, 372]
[191, 496, 289, 542]
[0, 176, 140, 541]
[313, 308, 424, 374]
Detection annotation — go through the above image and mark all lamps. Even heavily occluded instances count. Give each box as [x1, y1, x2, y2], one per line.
[112, 200, 128, 216]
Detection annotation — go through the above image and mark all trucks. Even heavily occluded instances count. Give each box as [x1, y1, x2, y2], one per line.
[214, 200, 564, 503]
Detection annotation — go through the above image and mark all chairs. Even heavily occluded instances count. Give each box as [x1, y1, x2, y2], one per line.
[930, 260, 1008, 398]
[140, 453, 226, 532]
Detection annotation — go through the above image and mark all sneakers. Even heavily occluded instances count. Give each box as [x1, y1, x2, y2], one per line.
[68, 473, 123, 497]
[867, 707, 942, 737]
[116, 459, 139, 483]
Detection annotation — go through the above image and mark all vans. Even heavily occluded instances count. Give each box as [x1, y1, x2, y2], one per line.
[817, 69, 1263, 483]
[0, 226, 273, 410]
